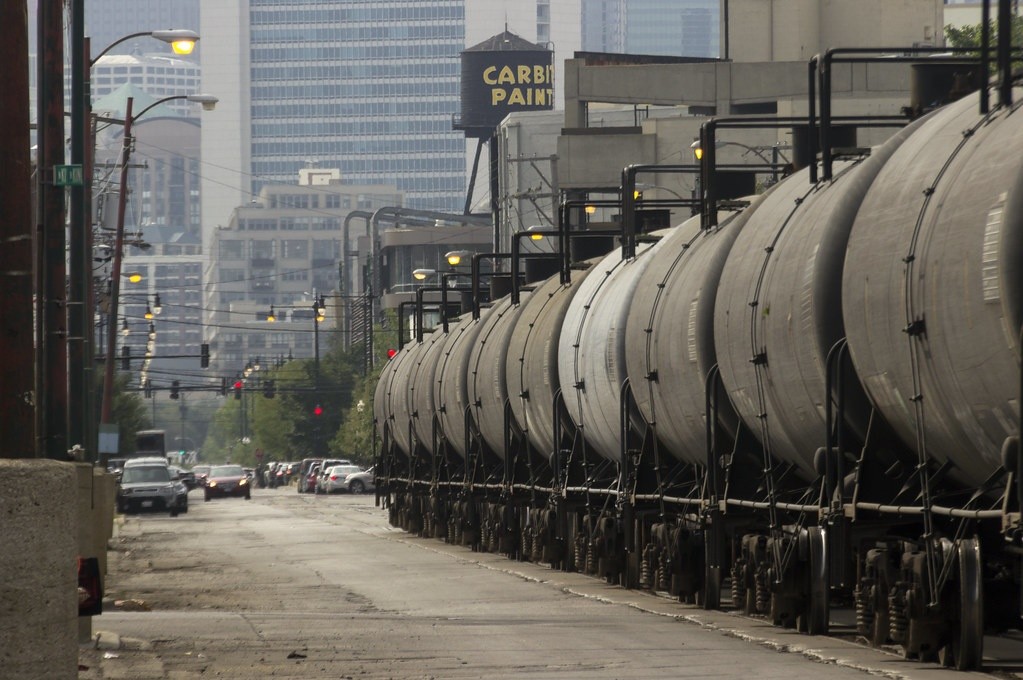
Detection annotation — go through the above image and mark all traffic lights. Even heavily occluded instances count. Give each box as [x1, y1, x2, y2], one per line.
[314, 404, 323, 416]
[233, 381, 242, 399]
[386, 348, 397, 359]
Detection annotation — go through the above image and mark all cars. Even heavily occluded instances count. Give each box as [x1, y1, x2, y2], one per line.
[325, 464, 361, 495]
[168, 467, 192, 514]
[320, 467, 335, 492]
[107, 428, 300, 492]
[305, 467, 322, 492]
[300, 462, 320, 492]
[343, 463, 378, 495]
[201, 465, 252, 503]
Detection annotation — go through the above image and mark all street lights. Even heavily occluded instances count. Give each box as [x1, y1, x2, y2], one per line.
[82, 29, 205, 466]
[268, 301, 323, 375]
[317, 291, 380, 378]
[95, 93, 218, 467]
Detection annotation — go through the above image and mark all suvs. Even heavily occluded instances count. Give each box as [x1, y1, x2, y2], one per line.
[314, 459, 351, 494]
[117, 456, 181, 517]
[297, 457, 322, 488]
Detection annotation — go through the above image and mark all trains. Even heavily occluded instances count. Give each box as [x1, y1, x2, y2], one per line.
[371, 1, 1023, 671]
